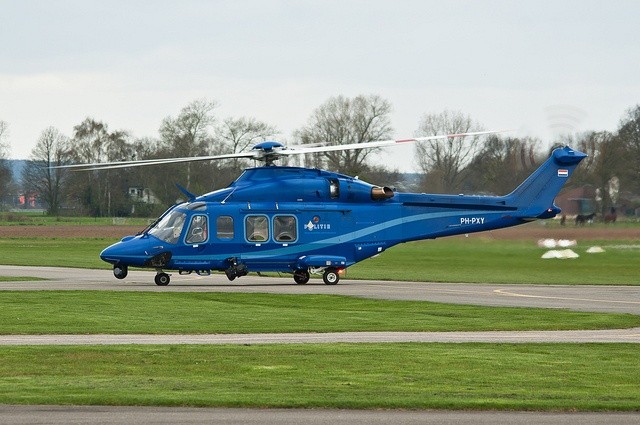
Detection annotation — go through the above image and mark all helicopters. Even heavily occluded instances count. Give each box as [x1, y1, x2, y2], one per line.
[40, 127, 589, 287]
